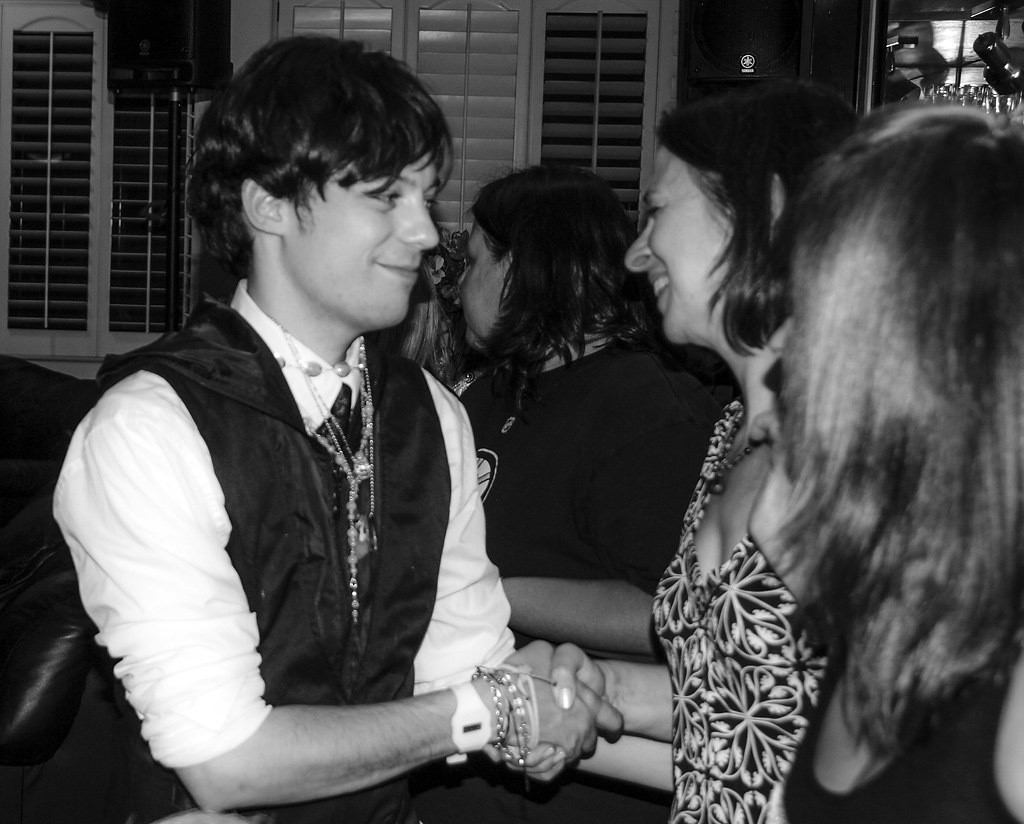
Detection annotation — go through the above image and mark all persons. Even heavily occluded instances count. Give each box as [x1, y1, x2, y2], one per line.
[450, 78, 859, 824]
[770, 108, 1024, 824]
[50, 34, 622, 824]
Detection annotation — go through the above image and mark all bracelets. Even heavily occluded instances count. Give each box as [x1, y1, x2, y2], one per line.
[469, 661, 540, 767]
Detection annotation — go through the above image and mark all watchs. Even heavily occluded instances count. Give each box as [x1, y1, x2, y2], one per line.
[448, 681, 492, 766]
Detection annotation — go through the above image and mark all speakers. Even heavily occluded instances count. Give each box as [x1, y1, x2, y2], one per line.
[106, 0, 232, 96]
[682, 0, 813, 103]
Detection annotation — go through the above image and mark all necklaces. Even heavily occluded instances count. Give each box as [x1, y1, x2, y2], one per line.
[263, 314, 378, 624]
[710, 406, 778, 497]
